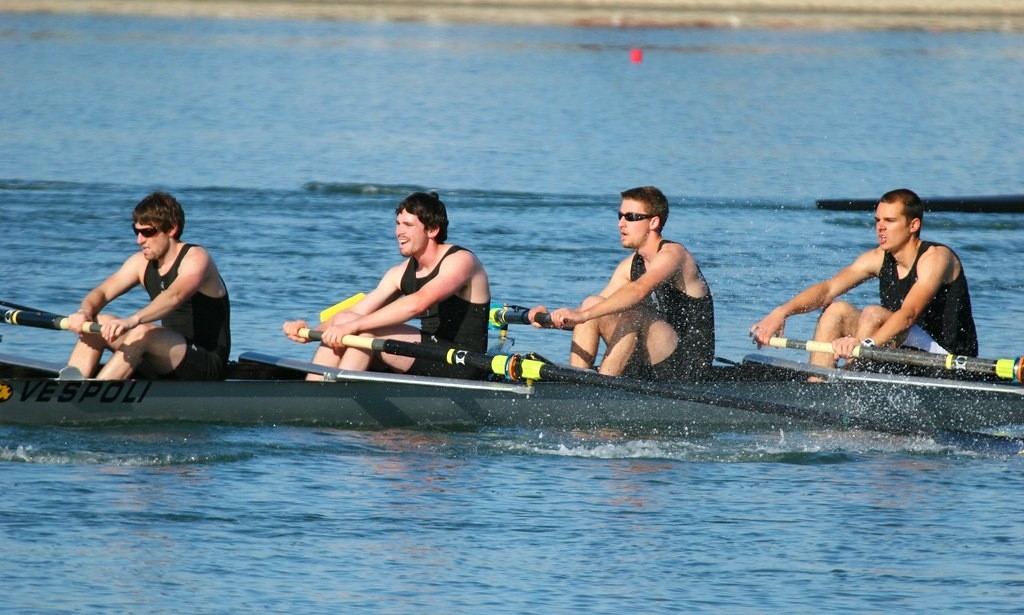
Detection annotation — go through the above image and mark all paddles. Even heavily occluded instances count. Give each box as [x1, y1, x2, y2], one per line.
[489, 305, 551, 328]
[299, 326, 1023, 442]
[768, 336, 1024, 384]
[1, 306, 102, 336]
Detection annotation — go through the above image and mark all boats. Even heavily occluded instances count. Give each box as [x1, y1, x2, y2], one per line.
[0, 337, 1024, 433]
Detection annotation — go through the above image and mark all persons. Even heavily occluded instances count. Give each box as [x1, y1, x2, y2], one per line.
[528, 187, 716, 381]
[750, 189, 978, 383]
[67, 192, 231, 381]
[285, 192, 490, 381]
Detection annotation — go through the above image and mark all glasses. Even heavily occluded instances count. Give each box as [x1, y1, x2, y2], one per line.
[618, 210, 658, 221]
[131, 221, 168, 237]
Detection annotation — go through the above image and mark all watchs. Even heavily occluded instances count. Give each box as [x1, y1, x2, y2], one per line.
[862, 338, 876, 349]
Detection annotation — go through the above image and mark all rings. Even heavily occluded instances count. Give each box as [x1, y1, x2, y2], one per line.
[760, 328, 763, 332]
